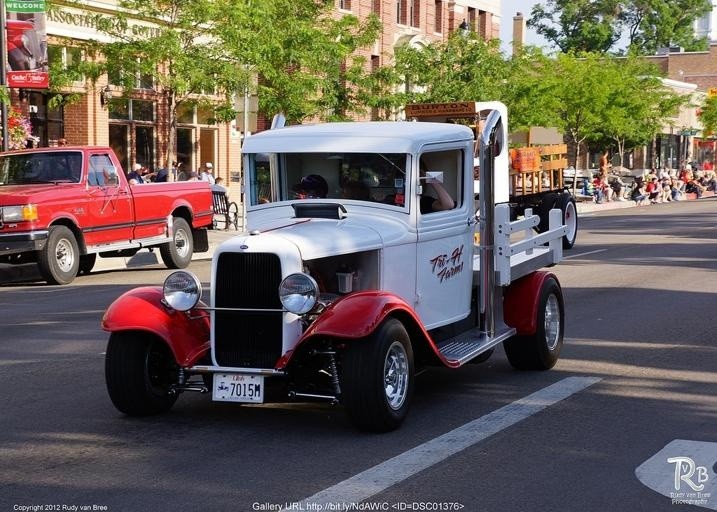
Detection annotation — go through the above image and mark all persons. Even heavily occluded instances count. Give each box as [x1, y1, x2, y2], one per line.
[16, 138, 69, 178]
[126, 160, 230, 210]
[580, 147, 717, 205]
[294, 157, 456, 215]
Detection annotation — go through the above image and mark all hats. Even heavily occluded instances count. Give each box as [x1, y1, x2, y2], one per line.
[291, 174, 329, 195]
[205, 162, 214, 169]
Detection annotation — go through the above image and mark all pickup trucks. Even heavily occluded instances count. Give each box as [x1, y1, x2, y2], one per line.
[0, 144, 217, 287]
